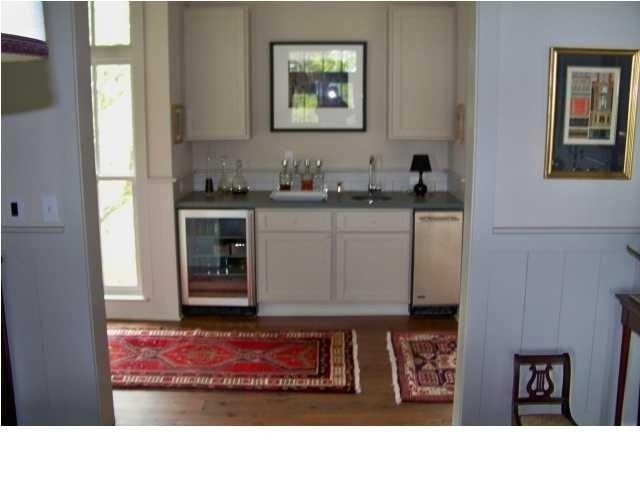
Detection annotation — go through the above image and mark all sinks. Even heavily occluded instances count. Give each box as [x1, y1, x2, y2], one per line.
[352, 194, 393, 201]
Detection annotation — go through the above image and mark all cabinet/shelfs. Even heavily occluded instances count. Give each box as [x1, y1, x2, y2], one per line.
[387, 4, 458, 143]
[256, 208, 412, 319]
[181, 5, 252, 142]
[2, 2, 51, 64]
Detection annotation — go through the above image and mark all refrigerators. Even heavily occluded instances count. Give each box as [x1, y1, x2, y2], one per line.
[176, 208, 257, 319]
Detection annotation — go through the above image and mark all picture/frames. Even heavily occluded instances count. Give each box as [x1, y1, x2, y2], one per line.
[543, 46, 639, 181]
[171, 104, 185, 145]
[269, 40, 367, 132]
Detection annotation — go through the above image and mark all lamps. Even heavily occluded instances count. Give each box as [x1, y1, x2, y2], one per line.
[410, 154, 433, 201]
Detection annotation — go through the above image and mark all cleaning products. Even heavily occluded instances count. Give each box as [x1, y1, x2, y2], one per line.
[280, 157, 325, 191]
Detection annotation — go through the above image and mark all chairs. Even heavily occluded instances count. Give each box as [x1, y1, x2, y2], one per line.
[511, 352, 580, 426]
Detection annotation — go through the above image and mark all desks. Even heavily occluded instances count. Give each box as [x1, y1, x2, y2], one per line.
[613, 292, 640, 426]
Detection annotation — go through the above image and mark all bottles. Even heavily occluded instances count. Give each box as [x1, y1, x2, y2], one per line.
[217, 160, 231, 193]
[277, 158, 326, 193]
[204, 155, 215, 194]
[232, 159, 249, 195]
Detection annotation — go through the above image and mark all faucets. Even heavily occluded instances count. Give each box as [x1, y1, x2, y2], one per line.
[368, 156, 376, 193]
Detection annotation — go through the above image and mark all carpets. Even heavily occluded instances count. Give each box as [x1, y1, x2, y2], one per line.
[385, 330, 459, 405]
[106, 327, 360, 396]
